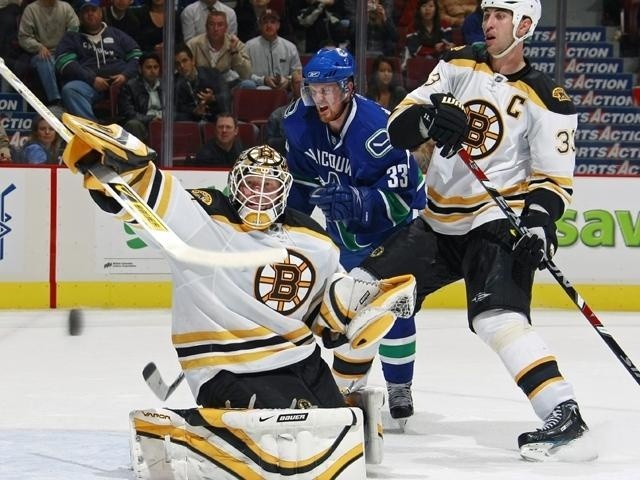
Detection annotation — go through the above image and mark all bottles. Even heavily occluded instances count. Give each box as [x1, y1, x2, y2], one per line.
[260, 9, 280, 22]
[80, 0, 99, 8]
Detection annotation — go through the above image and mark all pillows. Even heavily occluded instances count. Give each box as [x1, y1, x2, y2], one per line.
[481, 0, 542, 41]
[303, 48, 356, 90]
[228, 144, 293, 230]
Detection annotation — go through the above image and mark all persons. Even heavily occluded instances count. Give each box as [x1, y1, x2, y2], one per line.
[57, 111, 417, 480]
[331, 0, 598, 451]
[1, 0, 486, 172]
[280, 47, 428, 421]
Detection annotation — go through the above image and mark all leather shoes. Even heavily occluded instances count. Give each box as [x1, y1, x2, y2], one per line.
[422, 93, 467, 160]
[309, 185, 354, 221]
[513, 187, 565, 269]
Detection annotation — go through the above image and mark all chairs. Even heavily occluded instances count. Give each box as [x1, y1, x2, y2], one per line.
[518, 400, 589, 447]
[0, 0, 471, 171]
[387, 380, 412, 417]
